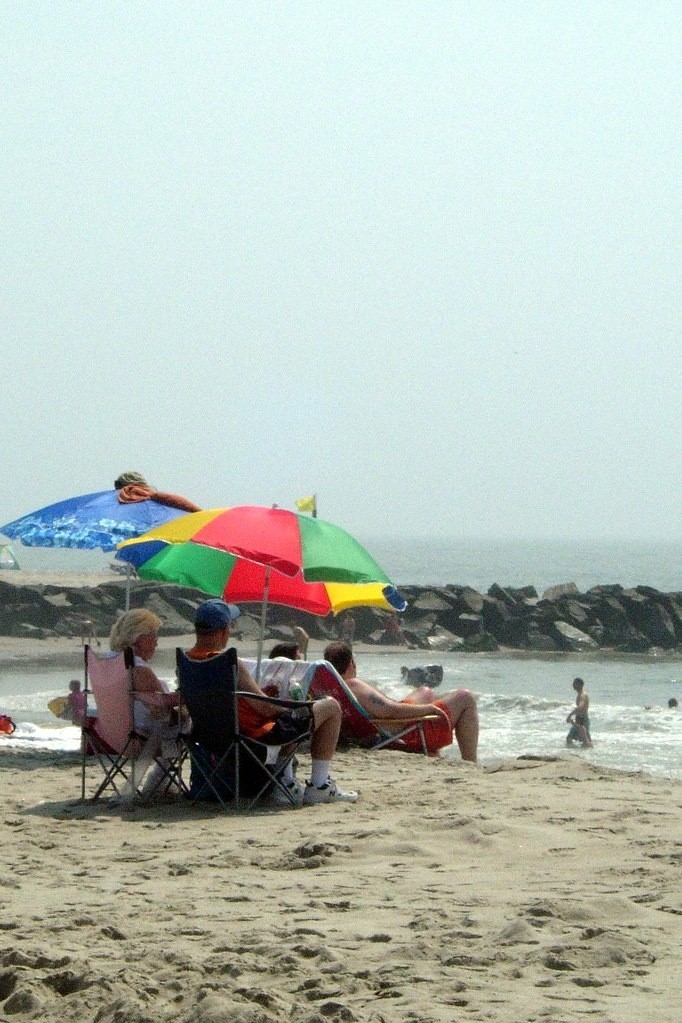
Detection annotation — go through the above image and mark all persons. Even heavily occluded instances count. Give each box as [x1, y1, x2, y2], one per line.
[56, 680, 85, 720]
[566, 678, 594, 748]
[110, 608, 190, 809]
[668, 698, 677, 708]
[183, 599, 359, 805]
[269, 643, 479, 762]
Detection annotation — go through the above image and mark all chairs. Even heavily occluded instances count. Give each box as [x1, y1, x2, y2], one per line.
[260, 659, 442, 757]
[82, 646, 190, 810]
[174, 647, 317, 816]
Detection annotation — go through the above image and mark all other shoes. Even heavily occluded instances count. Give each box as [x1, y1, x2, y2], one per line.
[143, 772, 178, 794]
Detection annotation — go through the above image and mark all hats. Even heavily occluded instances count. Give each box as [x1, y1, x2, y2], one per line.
[194, 599, 240, 634]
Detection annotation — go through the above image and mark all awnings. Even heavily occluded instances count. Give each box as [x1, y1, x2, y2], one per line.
[115, 504, 407, 690]
[0, 473, 205, 610]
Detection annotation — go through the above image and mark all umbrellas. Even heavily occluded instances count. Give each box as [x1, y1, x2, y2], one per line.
[47, 697, 67, 715]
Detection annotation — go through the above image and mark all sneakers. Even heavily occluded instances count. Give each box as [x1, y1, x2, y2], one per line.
[303, 774, 358, 805]
[270, 778, 307, 805]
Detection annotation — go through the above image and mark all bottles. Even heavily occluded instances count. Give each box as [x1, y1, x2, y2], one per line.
[290, 679, 304, 712]
